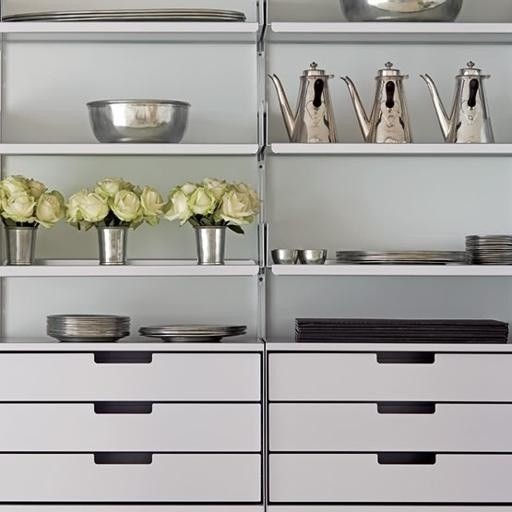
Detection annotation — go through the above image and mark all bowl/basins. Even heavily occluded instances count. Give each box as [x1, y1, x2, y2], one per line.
[272, 247, 299, 264]
[339, 1, 463, 22]
[85, 97, 192, 143]
[299, 249, 327, 264]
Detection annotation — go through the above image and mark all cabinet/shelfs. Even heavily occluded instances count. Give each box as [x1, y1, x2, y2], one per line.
[266, 0, 512, 512]
[0, 0, 265, 512]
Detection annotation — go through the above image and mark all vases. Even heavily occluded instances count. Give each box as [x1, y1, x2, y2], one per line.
[4, 225, 37, 266]
[97, 226, 129, 265]
[195, 226, 226, 265]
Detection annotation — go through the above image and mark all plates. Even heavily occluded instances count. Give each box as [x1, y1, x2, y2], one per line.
[335, 249, 472, 264]
[44, 312, 248, 343]
[1, 7, 247, 23]
[464, 232, 511, 265]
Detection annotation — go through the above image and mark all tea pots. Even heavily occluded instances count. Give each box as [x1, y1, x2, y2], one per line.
[418, 60, 495, 144]
[341, 60, 412, 143]
[268, 60, 338, 143]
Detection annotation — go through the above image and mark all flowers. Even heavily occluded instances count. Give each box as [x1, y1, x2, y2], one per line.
[164, 176, 260, 234]
[0, 176, 65, 229]
[64, 176, 165, 232]
